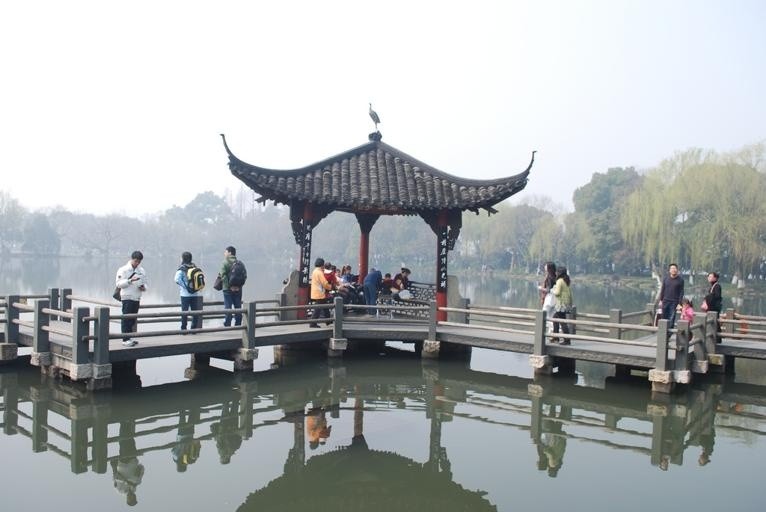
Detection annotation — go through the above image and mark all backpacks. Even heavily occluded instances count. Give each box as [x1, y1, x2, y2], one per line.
[177, 265, 204, 294]
[223, 260, 246, 286]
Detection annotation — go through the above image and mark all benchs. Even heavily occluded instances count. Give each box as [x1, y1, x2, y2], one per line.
[376, 294, 430, 306]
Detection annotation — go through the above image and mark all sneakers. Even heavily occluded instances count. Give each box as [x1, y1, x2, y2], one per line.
[122, 339, 138, 346]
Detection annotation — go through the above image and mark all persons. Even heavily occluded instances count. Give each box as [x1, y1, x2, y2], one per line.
[170, 407, 198, 473]
[547, 266, 574, 345]
[111, 419, 144, 507]
[173, 252, 200, 336]
[115, 250, 148, 348]
[531, 404, 567, 477]
[702, 271, 722, 344]
[323, 261, 412, 317]
[660, 263, 684, 342]
[679, 299, 695, 321]
[217, 246, 243, 327]
[699, 433, 716, 467]
[309, 258, 333, 328]
[536, 261, 556, 304]
[534, 403, 557, 470]
[305, 383, 332, 450]
[209, 400, 239, 464]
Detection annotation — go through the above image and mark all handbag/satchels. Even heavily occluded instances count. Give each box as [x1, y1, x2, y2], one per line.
[214, 273, 222, 290]
[700, 299, 708, 311]
[113, 288, 121, 301]
[571, 305, 576, 314]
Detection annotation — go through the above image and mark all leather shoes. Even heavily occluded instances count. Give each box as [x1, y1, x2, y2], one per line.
[310, 321, 332, 328]
[549, 338, 571, 345]
